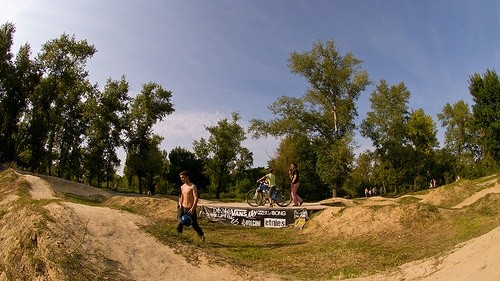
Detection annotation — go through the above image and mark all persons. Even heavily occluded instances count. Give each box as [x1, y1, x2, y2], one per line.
[253, 169, 277, 207]
[177, 171, 205, 244]
[429, 179, 436, 189]
[289, 163, 304, 206]
[365, 187, 375, 198]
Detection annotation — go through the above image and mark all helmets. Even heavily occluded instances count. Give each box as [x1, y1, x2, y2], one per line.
[181, 214, 193, 227]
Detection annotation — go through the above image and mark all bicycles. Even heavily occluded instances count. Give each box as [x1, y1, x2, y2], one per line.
[246, 180, 292, 207]
